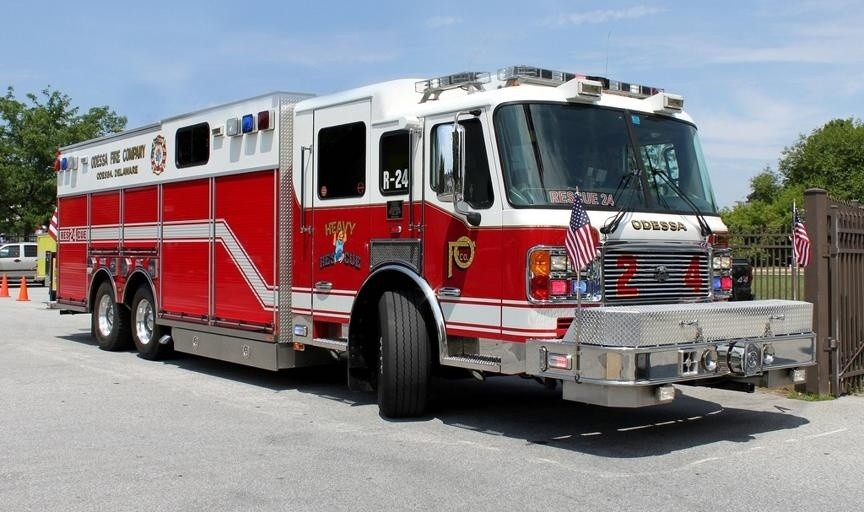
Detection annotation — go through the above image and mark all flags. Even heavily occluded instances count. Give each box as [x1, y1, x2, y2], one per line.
[561, 190, 597, 275]
[46, 207, 58, 242]
[790, 208, 812, 270]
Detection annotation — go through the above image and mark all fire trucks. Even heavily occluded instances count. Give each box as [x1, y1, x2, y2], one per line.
[44, 65, 818, 422]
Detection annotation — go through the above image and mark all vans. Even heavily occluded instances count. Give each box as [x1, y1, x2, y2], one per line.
[0, 242, 37, 283]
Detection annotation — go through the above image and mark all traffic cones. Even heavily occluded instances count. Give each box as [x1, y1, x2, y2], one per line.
[15, 275, 30, 301]
[0, 272, 11, 297]
